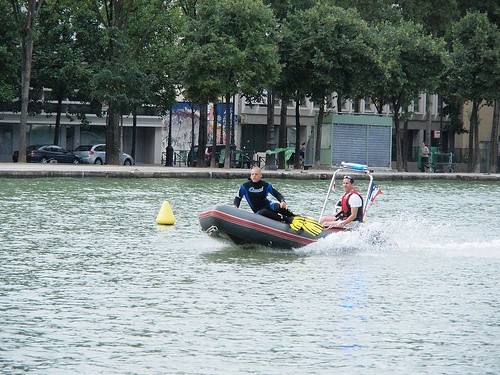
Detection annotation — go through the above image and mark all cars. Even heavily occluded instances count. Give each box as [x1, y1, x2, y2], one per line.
[72, 143, 135, 166]
[12, 144, 83, 165]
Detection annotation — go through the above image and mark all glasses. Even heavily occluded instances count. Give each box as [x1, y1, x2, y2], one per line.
[343, 176, 354, 181]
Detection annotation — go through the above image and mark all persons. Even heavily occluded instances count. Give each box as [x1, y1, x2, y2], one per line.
[233, 166, 295, 222]
[419, 142, 429, 172]
[300, 142, 306, 158]
[319, 176, 364, 228]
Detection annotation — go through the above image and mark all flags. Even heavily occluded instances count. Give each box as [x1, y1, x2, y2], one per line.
[368, 183, 381, 203]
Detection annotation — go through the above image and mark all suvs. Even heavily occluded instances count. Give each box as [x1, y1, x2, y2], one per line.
[186, 143, 260, 170]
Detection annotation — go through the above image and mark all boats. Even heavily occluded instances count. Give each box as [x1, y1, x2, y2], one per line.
[199, 162, 391, 258]
